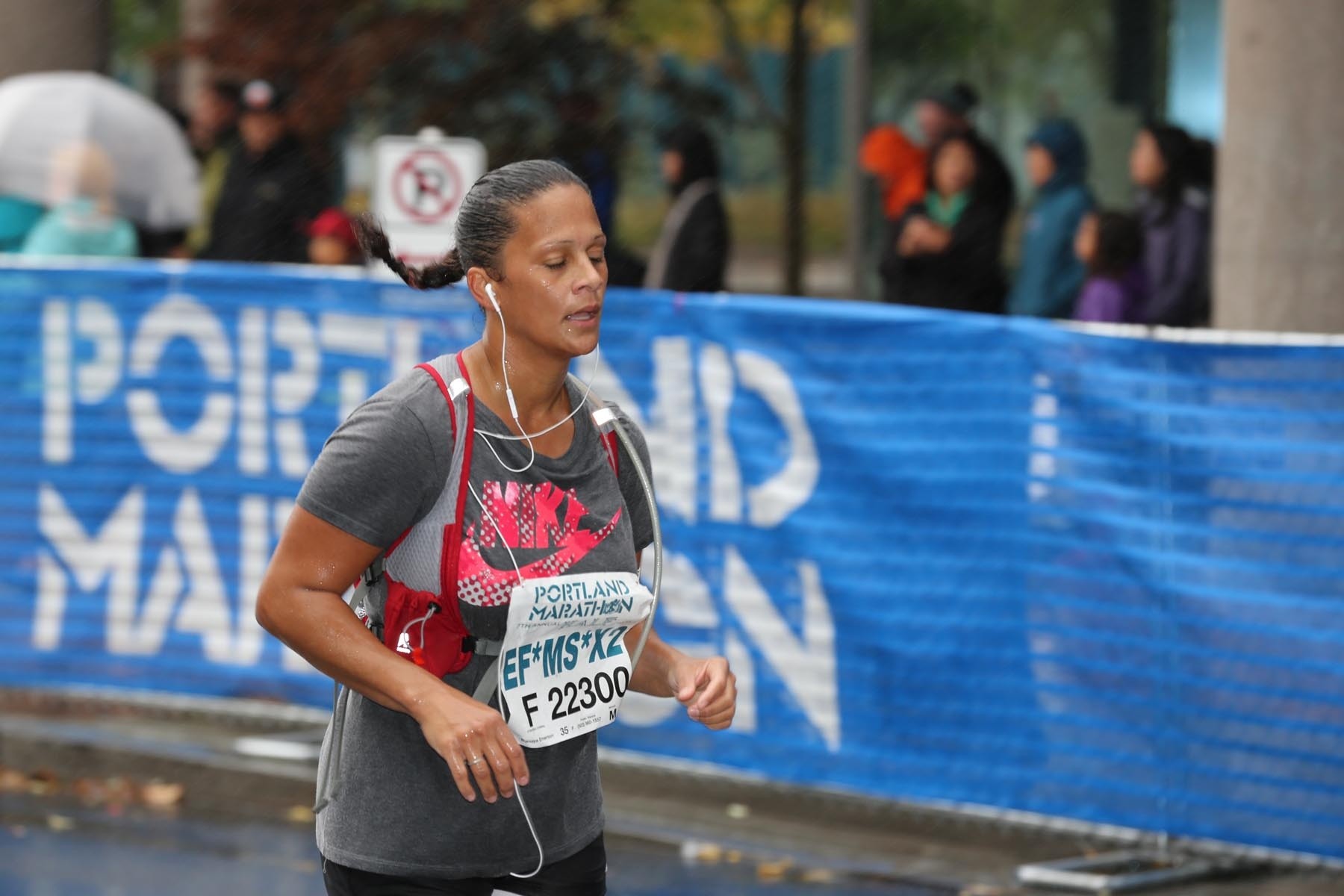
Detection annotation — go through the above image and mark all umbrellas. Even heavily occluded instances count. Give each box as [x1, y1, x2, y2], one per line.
[1, 70, 205, 230]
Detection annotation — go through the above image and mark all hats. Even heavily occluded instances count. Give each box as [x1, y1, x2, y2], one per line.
[219, 78, 289, 117]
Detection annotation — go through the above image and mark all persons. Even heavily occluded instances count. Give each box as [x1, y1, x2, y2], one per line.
[256, 160, 738, 895]
[0, 20, 1219, 336]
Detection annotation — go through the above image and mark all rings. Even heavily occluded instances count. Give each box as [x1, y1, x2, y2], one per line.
[468, 756, 486, 766]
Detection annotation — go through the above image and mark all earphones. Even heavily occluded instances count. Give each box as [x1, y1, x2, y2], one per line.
[485, 283, 503, 313]
